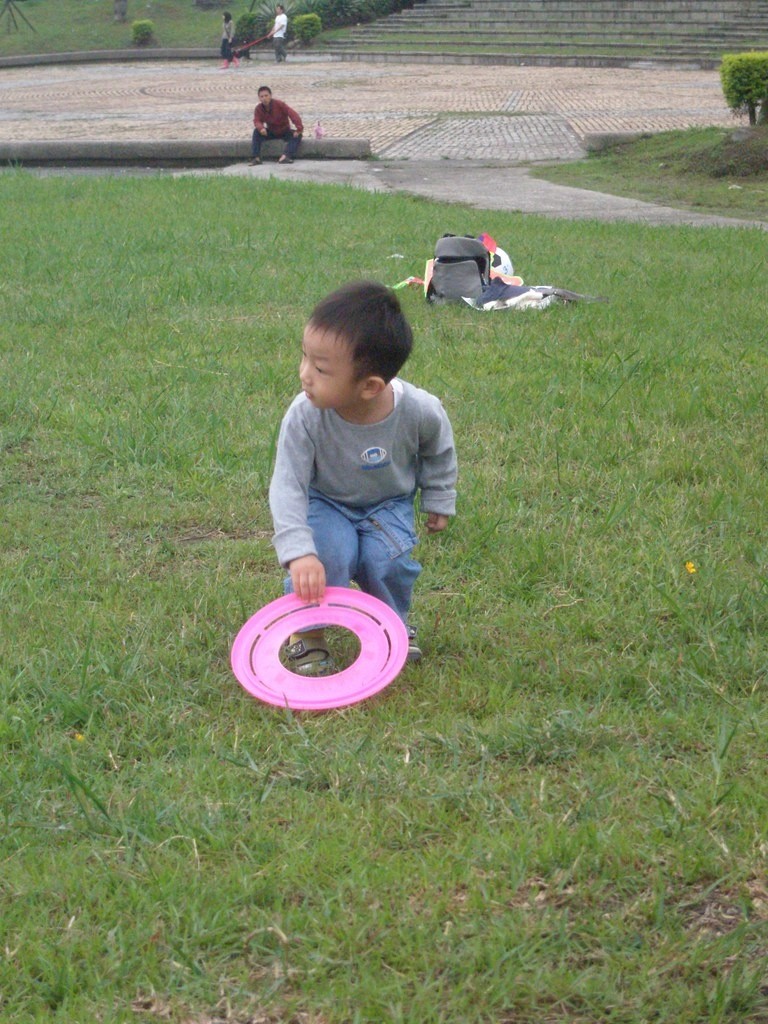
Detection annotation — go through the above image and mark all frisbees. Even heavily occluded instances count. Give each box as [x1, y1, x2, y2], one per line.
[230, 583, 411, 708]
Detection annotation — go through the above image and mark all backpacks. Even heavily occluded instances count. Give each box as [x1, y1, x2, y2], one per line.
[427, 233, 491, 307]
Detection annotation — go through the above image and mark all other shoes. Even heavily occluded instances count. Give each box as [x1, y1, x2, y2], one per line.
[286, 634, 339, 678]
[404, 624, 422, 662]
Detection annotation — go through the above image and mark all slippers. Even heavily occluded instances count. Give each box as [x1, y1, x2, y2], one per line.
[248, 159, 262, 167]
[278, 157, 293, 163]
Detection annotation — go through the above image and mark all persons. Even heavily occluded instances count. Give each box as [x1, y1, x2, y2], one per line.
[268, 5, 288, 64]
[219, 11, 240, 69]
[269, 281, 457, 674]
[247, 86, 304, 166]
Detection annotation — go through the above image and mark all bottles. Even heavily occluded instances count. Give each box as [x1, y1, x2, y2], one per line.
[316, 121, 322, 139]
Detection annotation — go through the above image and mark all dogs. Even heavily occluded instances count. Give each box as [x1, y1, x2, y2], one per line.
[232, 41, 252, 63]
